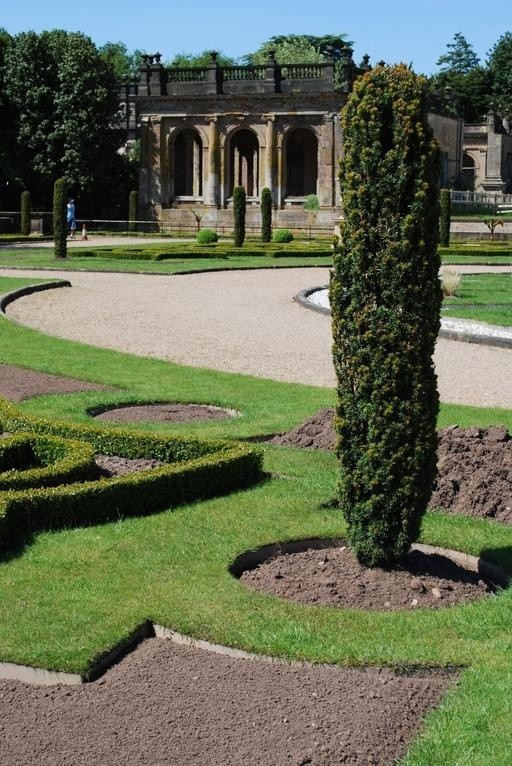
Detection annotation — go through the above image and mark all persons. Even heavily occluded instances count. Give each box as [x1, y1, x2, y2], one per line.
[67, 197, 77, 239]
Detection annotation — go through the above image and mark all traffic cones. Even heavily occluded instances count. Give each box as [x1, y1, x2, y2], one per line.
[78, 224, 91, 242]
